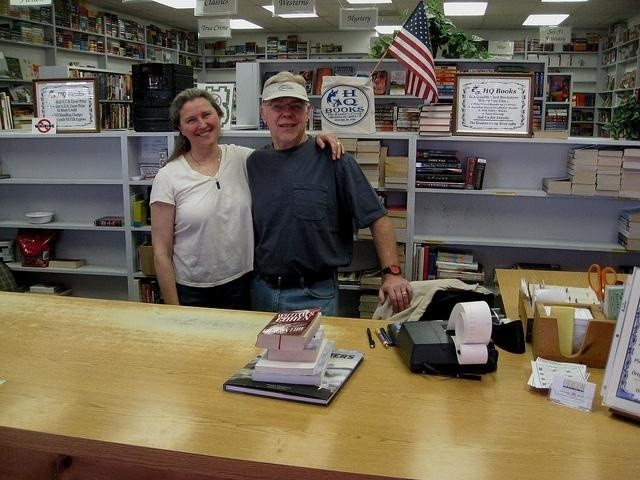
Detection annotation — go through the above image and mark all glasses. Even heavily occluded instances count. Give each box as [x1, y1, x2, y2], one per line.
[258, 103, 309, 112]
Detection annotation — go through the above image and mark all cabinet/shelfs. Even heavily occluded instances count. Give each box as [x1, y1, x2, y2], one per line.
[527, 40, 600, 136]
[128, 130, 412, 304]
[595, 37, 640, 138]
[235, 58, 574, 139]
[412, 132, 639, 306]
[55, 17, 105, 69]
[0, 129, 129, 301]
[204, 39, 266, 70]
[0, 0, 54, 66]
[38, 66, 133, 131]
[513, 36, 526, 59]
[146, 34, 178, 63]
[106, 27, 145, 71]
[266, 42, 308, 59]
[178, 50, 203, 71]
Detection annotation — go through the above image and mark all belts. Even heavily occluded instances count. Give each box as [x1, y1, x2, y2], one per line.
[251, 269, 337, 288]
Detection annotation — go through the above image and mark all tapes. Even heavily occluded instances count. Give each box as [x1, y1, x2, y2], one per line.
[492, 308, 512, 324]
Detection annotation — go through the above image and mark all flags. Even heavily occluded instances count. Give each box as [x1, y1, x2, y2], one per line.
[386, 0, 441, 105]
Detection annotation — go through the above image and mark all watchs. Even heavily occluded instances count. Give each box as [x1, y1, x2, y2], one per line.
[381, 264, 402, 276]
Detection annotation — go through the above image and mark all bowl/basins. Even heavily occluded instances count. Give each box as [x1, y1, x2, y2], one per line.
[24, 209, 55, 225]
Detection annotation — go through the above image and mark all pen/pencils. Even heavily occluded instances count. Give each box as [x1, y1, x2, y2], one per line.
[375, 327, 393, 348]
[367, 328, 375, 348]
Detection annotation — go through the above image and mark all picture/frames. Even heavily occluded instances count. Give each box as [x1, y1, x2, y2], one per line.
[32, 78, 101, 134]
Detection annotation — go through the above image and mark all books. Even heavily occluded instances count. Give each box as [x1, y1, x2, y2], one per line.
[358, 295, 381, 320]
[358, 191, 407, 240]
[94, 217, 125, 228]
[372, 71, 391, 95]
[0, 83, 36, 130]
[260, 99, 269, 130]
[205, 41, 265, 54]
[222, 308, 366, 407]
[397, 100, 454, 137]
[106, 13, 145, 42]
[415, 149, 465, 190]
[338, 138, 388, 190]
[532, 72, 570, 132]
[413, 242, 485, 286]
[598, 109, 611, 122]
[267, 35, 308, 53]
[178, 31, 199, 54]
[356, 72, 369, 78]
[337, 267, 385, 291]
[179, 52, 203, 68]
[0, 0, 53, 24]
[316, 68, 332, 95]
[334, 66, 354, 76]
[601, 267, 640, 422]
[474, 157, 487, 188]
[300, 70, 313, 94]
[602, 40, 640, 66]
[98, 102, 135, 131]
[0, 19, 55, 47]
[374, 103, 398, 133]
[600, 21, 640, 50]
[56, 27, 105, 52]
[310, 43, 342, 53]
[205, 55, 265, 68]
[68, 61, 99, 80]
[390, 70, 405, 96]
[513, 32, 600, 53]
[267, 55, 307, 59]
[47, 259, 86, 269]
[469, 66, 526, 74]
[617, 207, 640, 251]
[543, 144, 640, 198]
[605, 69, 640, 90]
[571, 93, 597, 107]
[107, 38, 145, 60]
[145, 24, 177, 50]
[147, 46, 177, 63]
[601, 94, 613, 107]
[305, 105, 322, 131]
[572, 109, 594, 121]
[91, 72, 132, 101]
[55, 0, 105, 34]
[599, 125, 626, 139]
[570, 123, 594, 137]
[25, 282, 72, 297]
[396, 243, 405, 273]
[385, 153, 408, 189]
[528, 55, 581, 68]
[0, 49, 43, 79]
[433, 66, 456, 100]
[465, 156, 478, 189]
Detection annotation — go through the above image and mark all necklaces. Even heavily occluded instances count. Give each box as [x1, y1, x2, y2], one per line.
[188, 145, 221, 191]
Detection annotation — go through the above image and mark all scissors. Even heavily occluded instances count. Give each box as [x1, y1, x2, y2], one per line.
[588, 264, 617, 312]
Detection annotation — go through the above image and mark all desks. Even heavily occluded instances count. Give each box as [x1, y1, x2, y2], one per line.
[0, 272, 640, 479]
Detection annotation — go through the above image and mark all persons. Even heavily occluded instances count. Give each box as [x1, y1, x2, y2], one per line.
[246, 71, 413, 317]
[149, 87, 345, 311]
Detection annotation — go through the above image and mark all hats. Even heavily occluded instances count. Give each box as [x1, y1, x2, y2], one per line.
[262, 71, 310, 102]
